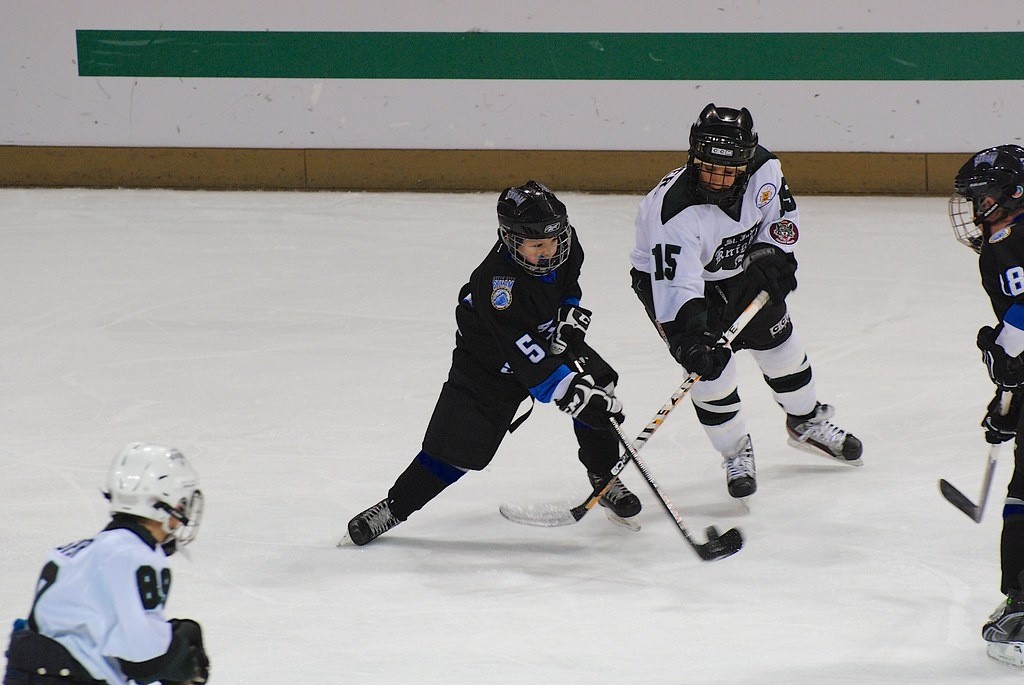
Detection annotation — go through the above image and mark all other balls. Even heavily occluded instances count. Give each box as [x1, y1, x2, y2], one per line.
[705, 525, 719, 541]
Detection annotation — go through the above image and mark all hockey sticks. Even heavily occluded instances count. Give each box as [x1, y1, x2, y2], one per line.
[572, 358, 745, 562]
[938, 390, 1015, 525]
[497, 287, 771, 527]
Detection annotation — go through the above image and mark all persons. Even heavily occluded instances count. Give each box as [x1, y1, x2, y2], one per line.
[336, 181, 643, 545]
[628, 102, 865, 513]
[1, 442, 210, 685]
[948, 144, 1024, 668]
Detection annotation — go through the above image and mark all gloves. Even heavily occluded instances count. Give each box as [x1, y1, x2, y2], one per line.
[981, 387, 1024, 444]
[668, 312, 732, 383]
[549, 304, 592, 368]
[741, 243, 798, 308]
[160, 618, 210, 685]
[977, 322, 1024, 395]
[554, 373, 625, 430]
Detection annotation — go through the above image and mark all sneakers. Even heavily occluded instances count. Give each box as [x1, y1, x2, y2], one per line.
[579, 448, 642, 531]
[335, 499, 403, 548]
[981, 592, 1024, 670]
[721, 434, 758, 513]
[786, 402, 864, 467]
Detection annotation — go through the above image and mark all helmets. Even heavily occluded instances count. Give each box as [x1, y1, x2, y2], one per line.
[687, 102, 759, 205]
[496, 180, 572, 276]
[948, 144, 1024, 255]
[107, 441, 201, 522]
